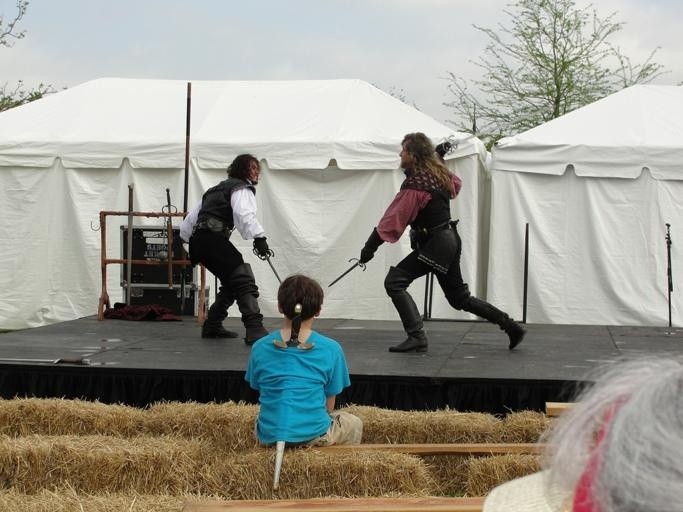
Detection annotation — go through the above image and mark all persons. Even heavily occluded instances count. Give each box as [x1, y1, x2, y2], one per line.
[171, 153, 270, 346]
[244, 274, 364, 449]
[482, 352, 683, 512]
[359, 131, 529, 353]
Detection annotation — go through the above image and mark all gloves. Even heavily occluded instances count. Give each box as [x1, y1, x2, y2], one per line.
[436, 142, 451, 158]
[360, 227, 385, 264]
[254, 236, 269, 257]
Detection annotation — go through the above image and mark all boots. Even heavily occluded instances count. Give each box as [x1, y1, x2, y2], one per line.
[228, 262, 269, 345]
[387, 291, 429, 354]
[201, 282, 240, 339]
[463, 296, 527, 351]
